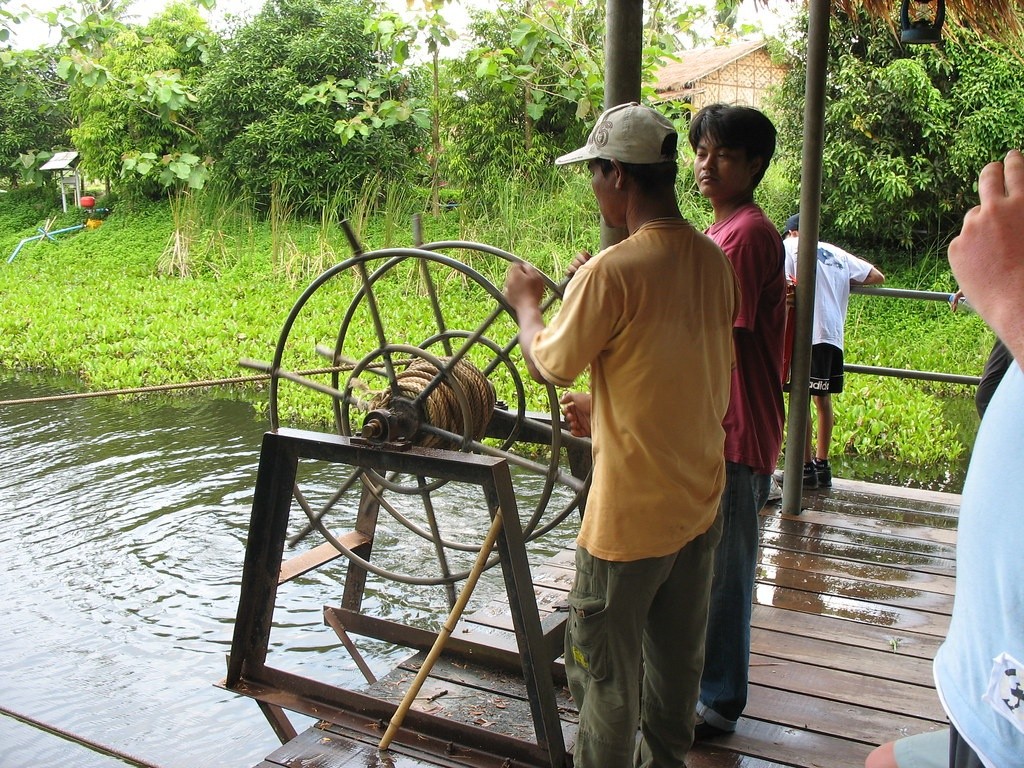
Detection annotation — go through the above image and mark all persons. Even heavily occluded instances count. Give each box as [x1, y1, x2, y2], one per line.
[688, 104, 787, 735]
[773, 212, 885, 489]
[865, 148, 1024, 768]
[505, 102, 743, 768]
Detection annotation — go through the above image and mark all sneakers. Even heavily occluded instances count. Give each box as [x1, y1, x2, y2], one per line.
[811, 457, 832, 487]
[695, 713, 725, 742]
[774, 462, 818, 490]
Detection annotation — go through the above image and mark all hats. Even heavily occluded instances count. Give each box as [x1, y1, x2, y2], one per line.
[781, 213, 799, 240]
[555, 102, 679, 166]
